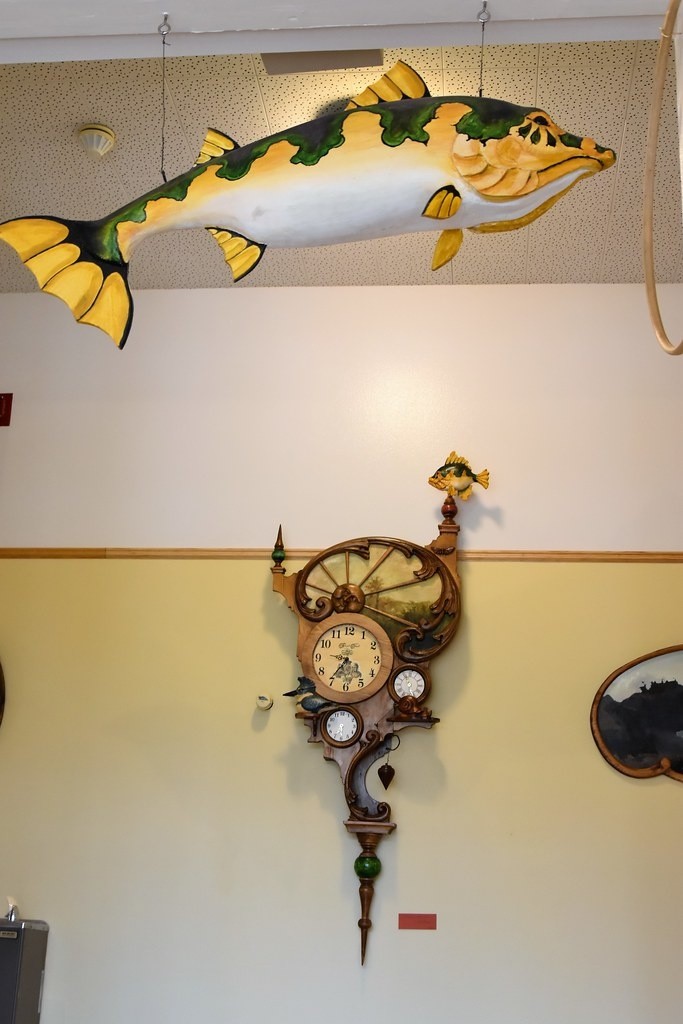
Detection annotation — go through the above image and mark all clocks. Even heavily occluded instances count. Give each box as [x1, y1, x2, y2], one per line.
[301, 612, 393, 704]
[387, 664, 430, 703]
[320, 705, 363, 749]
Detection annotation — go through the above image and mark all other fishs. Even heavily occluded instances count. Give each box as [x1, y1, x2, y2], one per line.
[0, 61, 618, 351]
